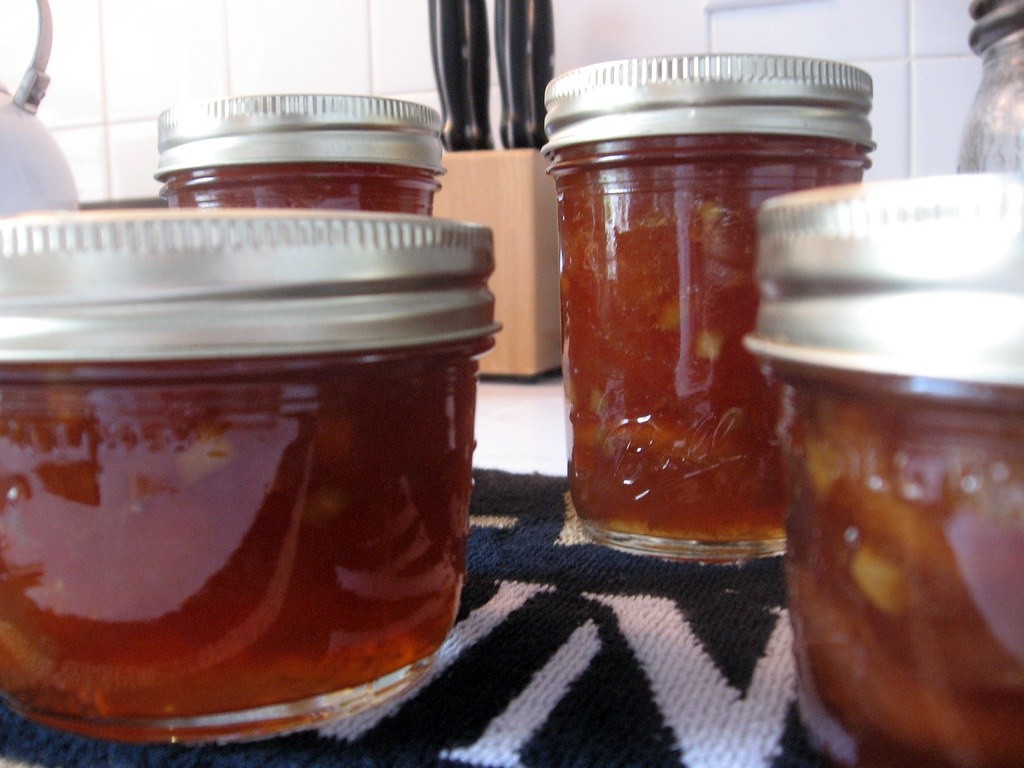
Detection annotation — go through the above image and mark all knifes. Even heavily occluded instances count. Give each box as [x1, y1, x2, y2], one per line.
[411, 2, 559, 376]
